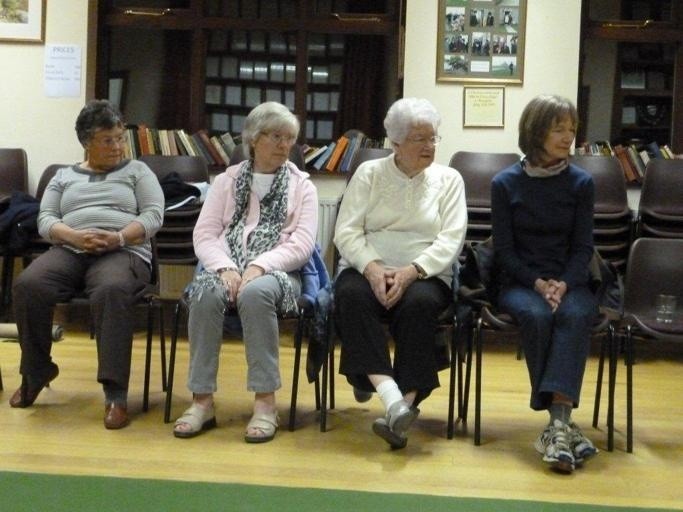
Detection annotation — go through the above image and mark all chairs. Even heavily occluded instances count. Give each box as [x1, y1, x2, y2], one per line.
[36, 165, 167, 411]
[319, 149, 465, 439]
[570, 154, 637, 276]
[163, 144, 320, 431]
[0, 147, 31, 316]
[136, 154, 210, 266]
[461, 304, 618, 452]
[447, 149, 521, 265]
[607, 238, 683, 453]
[638, 157, 683, 239]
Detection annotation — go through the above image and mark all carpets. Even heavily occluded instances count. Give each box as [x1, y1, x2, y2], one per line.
[0, 462, 683, 512]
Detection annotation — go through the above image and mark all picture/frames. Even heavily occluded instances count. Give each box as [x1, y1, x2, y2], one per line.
[0, 0, 47, 45]
[436, 0, 528, 87]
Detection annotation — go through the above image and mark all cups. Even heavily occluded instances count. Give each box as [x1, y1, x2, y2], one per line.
[655, 294, 676, 323]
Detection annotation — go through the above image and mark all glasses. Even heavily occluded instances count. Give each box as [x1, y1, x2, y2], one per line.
[95, 134, 128, 145]
[260, 131, 292, 144]
[407, 133, 442, 146]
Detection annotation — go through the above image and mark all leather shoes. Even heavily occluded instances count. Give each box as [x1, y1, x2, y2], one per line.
[103, 395, 128, 429]
[10, 364, 60, 407]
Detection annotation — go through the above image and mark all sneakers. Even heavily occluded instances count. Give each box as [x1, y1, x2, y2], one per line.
[534, 418, 575, 472]
[568, 422, 599, 464]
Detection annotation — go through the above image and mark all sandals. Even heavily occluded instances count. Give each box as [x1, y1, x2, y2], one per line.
[372, 418, 408, 448]
[173, 406, 217, 438]
[244, 409, 279, 443]
[385, 400, 420, 436]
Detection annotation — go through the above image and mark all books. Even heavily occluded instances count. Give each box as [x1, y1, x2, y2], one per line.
[118, 122, 396, 176]
[576, 139, 681, 187]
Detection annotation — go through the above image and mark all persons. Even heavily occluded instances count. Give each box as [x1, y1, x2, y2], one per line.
[9, 101, 167, 432]
[330, 97, 470, 451]
[442, 8, 520, 78]
[486, 94, 601, 477]
[172, 100, 321, 446]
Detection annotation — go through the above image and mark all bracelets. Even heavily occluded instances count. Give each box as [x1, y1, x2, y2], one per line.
[410, 260, 428, 277]
[115, 228, 127, 250]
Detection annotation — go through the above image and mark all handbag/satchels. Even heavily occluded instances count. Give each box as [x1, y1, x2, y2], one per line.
[158, 171, 209, 212]
